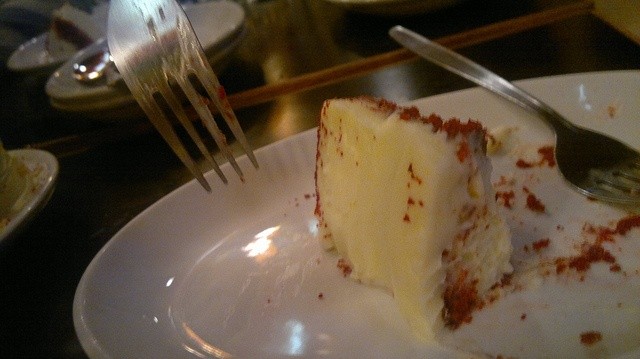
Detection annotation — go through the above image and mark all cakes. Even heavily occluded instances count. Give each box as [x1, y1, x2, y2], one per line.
[314, 95, 513, 339]
[0, 148, 42, 228]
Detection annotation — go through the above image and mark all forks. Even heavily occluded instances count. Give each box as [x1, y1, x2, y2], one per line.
[388, 25, 640, 205]
[105, 0, 260, 192]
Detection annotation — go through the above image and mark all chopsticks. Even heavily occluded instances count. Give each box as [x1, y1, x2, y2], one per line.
[180, 1, 593, 124]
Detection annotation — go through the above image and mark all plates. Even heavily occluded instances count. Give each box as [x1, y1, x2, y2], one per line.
[50, 16, 251, 114]
[73, 70, 639, 358]
[7, 15, 108, 71]
[1, 149, 59, 241]
[44, 0, 246, 101]
